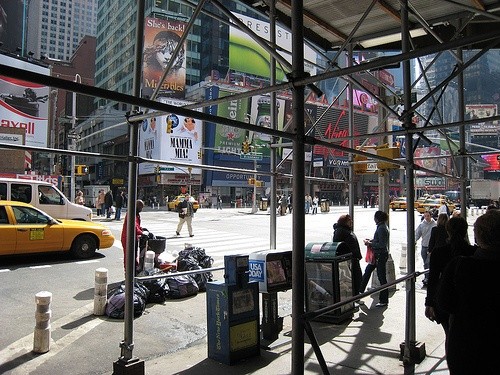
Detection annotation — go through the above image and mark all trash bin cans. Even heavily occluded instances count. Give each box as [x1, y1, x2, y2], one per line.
[320, 199, 329, 212]
[259, 197, 268, 210]
[304, 242, 359, 325]
[139, 235, 167, 272]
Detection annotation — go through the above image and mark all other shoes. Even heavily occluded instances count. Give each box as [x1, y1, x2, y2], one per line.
[176, 231, 180, 235]
[190, 235, 194, 237]
[375, 302, 388, 306]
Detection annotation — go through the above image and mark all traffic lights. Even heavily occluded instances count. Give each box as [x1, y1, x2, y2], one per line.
[242, 141, 249, 154]
[197, 151, 202, 159]
[154, 166, 160, 175]
[82, 168, 89, 173]
[250, 179, 255, 184]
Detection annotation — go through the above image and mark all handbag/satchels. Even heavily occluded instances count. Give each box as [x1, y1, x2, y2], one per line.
[108, 206, 116, 213]
[365, 246, 375, 265]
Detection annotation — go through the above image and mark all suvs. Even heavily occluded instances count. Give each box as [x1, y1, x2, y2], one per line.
[0, 178, 93, 222]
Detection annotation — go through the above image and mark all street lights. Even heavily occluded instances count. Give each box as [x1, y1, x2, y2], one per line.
[244, 113, 257, 214]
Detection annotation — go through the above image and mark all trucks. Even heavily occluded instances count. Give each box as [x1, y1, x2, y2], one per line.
[470, 179, 500, 208]
[82, 185, 111, 208]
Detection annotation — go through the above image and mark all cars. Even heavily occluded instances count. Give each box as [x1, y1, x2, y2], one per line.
[0, 200, 115, 260]
[389, 191, 462, 215]
[168, 196, 199, 213]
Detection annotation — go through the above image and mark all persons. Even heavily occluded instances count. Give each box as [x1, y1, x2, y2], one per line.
[409, 194, 466, 288]
[94, 187, 128, 221]
[424, 216, 478, 347]
[332, 214, 364, 306]
[76, 190, 87, 206]
[275, 192, 319, 216]
[438, 209, 500, 375]
[358, 210, 390, 307]
[217, 195, 222, 209]
[145, 30, 185, 72]
[122, 199, 149, 281]
[176, 193, 195, 237]
